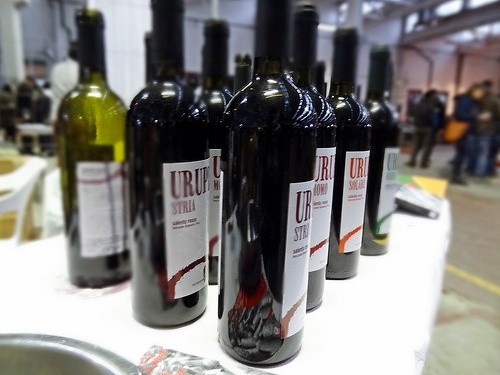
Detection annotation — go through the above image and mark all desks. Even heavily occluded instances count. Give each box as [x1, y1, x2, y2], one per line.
[0, 185, 451, 375]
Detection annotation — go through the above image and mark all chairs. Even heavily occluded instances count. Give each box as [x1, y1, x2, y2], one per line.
[15, 57, 81, 155]
[0, 157, 64, 244]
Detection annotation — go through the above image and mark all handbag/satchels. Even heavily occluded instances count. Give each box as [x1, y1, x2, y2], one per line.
[442, 116, 470, 143]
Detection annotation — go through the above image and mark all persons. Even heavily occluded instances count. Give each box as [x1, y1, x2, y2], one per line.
[449, 83, 486, 188]
[393, 88, 446, 170]
[468, 79, 500, 178]
[0, 74, 53, 157]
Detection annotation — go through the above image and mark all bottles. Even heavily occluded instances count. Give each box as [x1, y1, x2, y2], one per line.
[126, 32, 206, 327]
[229, 52, 253, 95]
[364, 43, 401, 256]
[194, 17, 235, 285]
[219, 0, 316, 368]
[55, 7, 133, 289]
[326, 26, 370, 279]
[291, 2, 337, 313]
